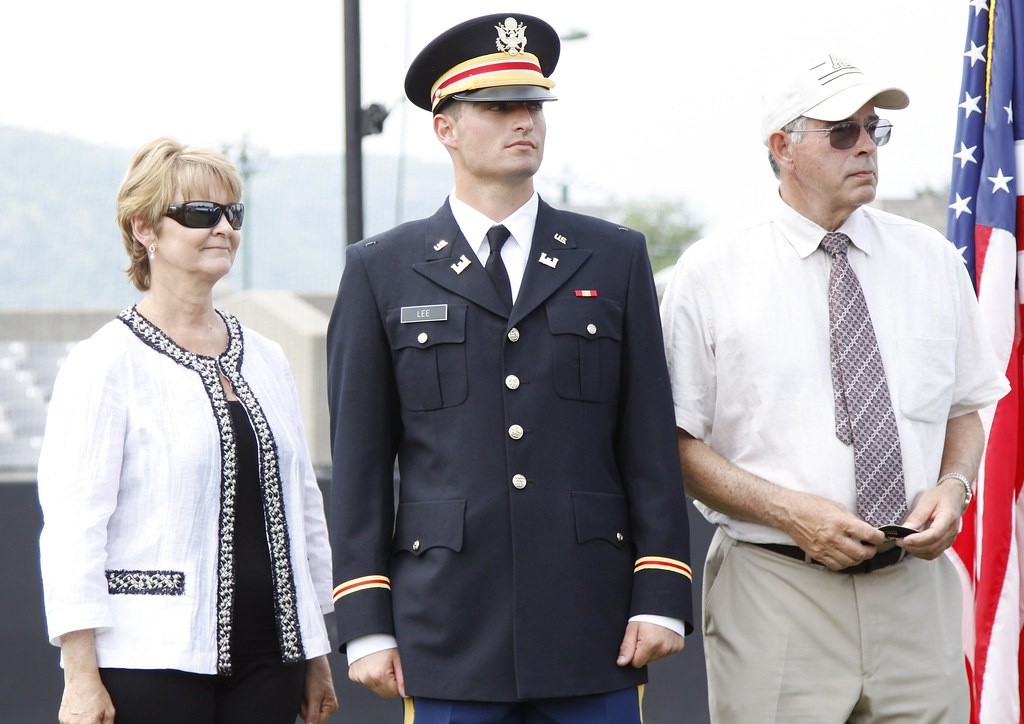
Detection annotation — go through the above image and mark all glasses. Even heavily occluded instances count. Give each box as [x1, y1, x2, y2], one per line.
[161, 200, 246, 231]
[785, 117, 893, 150]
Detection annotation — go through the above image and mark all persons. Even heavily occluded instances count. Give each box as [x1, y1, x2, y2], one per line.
[658, 55, 1012, 724]
[37, 139, 338, 724]
[327, 14, 694, 724]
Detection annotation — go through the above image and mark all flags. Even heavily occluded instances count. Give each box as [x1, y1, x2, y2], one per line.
[943, 0, 1024, 724]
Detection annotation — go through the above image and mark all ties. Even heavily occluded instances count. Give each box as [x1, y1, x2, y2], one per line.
[819, 232, 908, 528]
[484, 226, 513, 314]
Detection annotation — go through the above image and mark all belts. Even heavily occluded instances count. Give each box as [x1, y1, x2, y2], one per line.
[757, 538, 910, 575]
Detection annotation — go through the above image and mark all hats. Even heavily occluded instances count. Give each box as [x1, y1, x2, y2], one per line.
[763, 54, 909, 147]
[405, 13, 561, 117]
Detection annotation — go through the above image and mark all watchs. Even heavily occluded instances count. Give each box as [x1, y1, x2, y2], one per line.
[938, 473, 973, 514]
